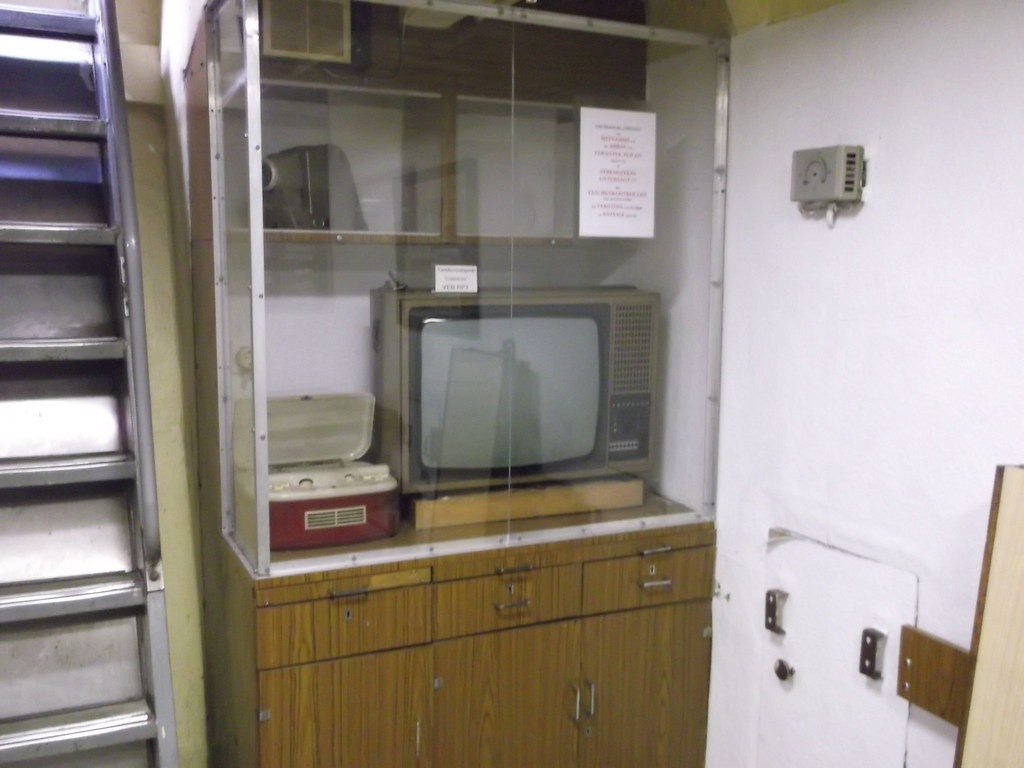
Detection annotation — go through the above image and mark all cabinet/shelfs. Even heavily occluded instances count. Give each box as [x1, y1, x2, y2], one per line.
[185, 0, 729, 767]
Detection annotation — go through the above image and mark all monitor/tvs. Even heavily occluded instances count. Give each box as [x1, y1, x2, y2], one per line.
[369, 287, 659, 500]
